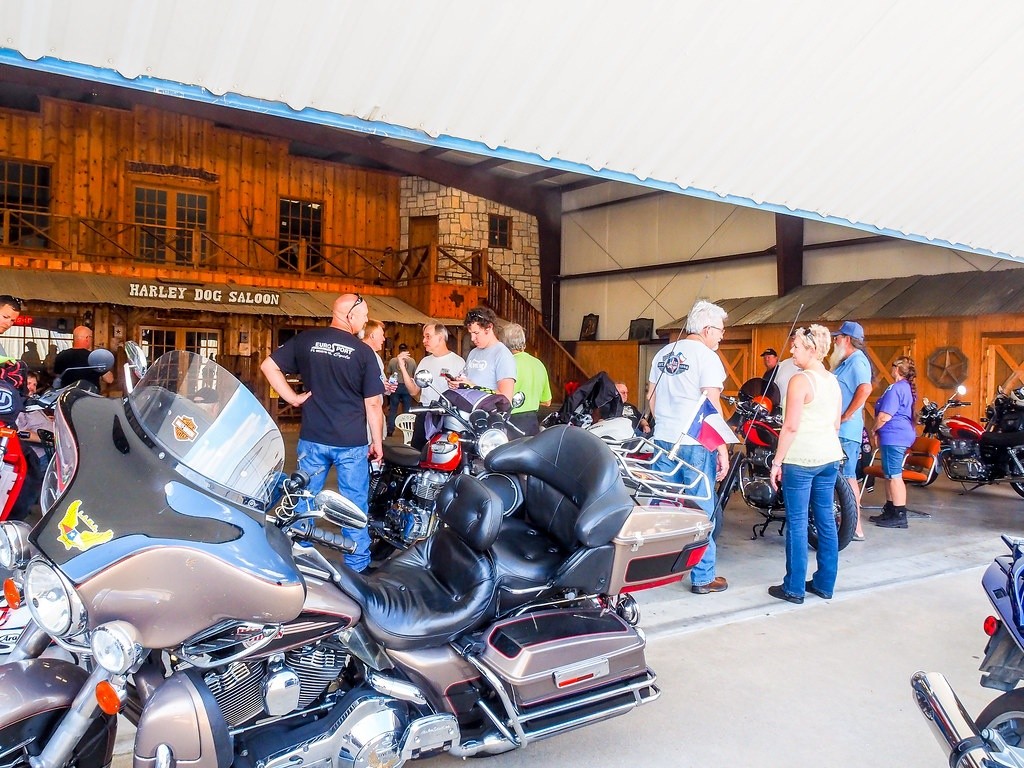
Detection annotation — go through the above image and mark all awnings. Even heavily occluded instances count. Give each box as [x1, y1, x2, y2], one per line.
[0, 265, 436, 325]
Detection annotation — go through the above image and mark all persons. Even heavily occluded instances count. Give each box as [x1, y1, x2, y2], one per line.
[0, 295, 114, 395]
[646, 301, 729, 594]
[867, 357, 917, 528]
[261, 293, 386, 575]
[612, 383, 653, 439]
[830, 321, 872, 542]
[362, 306, 552, 472]
[759, 326, 804, 410]
[768, 323, 844, 605]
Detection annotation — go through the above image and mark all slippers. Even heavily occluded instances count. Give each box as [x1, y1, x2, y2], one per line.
[851, 531, 865, 541]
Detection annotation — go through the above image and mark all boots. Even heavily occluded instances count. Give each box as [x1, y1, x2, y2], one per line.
[869, 501, 894, 522]
[875, 505, 908, 528]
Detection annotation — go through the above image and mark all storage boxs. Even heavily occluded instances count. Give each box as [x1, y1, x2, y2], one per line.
[476, 609, 648, 707]
[607, 464, 715, 596]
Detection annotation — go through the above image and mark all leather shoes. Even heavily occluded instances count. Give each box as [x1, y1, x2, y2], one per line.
[691, 576, 729, 594]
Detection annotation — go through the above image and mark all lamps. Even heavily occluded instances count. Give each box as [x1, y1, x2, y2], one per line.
[82, 309, 94, 330]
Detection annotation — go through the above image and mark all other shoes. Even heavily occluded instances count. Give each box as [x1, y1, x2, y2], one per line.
[355, 566, 378, 578]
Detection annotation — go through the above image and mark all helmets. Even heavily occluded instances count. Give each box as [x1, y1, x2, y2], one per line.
[1010, 386, 1024, 410]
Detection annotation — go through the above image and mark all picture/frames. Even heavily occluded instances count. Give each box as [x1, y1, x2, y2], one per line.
[628, 317, 654, 342]
[579, 313, 599, 341]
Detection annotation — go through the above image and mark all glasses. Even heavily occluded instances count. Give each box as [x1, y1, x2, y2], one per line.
[0, 293, 23, 305]
[346, 293, 364, 319]
[465, 311, 491, 323]
[801, 323, 817, 351]
[702, 325, 726, 335]
[891, 361, 897, 368]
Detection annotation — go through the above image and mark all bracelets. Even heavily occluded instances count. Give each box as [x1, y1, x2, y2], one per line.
[772, 459, 782, 467]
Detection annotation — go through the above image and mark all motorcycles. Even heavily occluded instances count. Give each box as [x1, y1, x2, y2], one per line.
[913, 533, 1024, 767]
[0, 343, 714, 768]
[916, 385, 1024, 499]
[720, 377, 858, 553]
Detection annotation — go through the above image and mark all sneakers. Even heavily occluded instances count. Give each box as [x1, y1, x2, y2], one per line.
[804, 579, 832, 599]
[768, 584, 804, 604]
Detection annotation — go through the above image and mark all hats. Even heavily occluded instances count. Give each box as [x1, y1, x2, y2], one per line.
[759, 348, 778, 357]
[830, 321, 865, 344]
[398, 343, 408, 350]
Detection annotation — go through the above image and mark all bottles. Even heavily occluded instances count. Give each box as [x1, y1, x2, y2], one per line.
[384, 372, 398, 395]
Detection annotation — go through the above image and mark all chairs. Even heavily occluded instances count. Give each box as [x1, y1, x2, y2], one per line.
[395, 414, 416, 446]
[474, 425, 630, 611]
[859, 436, 941, 518]
